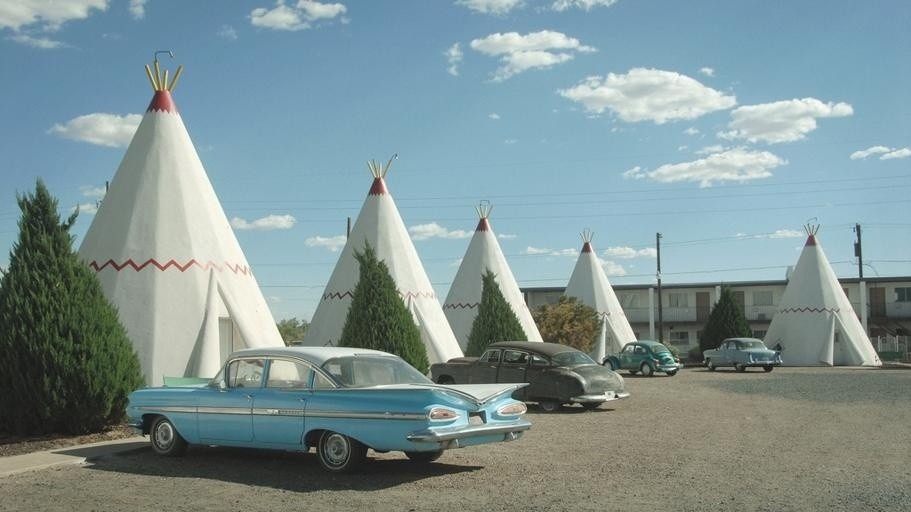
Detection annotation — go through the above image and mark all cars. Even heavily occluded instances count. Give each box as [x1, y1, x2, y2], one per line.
[703, 337, 785, 374]
[126, 346, 532, 473]
[427, 339, 631, 416]
[601, 340, 687, 378]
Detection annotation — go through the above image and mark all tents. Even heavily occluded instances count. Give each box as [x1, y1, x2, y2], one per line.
[290, 154, 466, 380]
[763, 223, 883, 367]
[563, 227, 636, 366]
[76, 49, 300, 389]
[440, 199, 543, 356]
